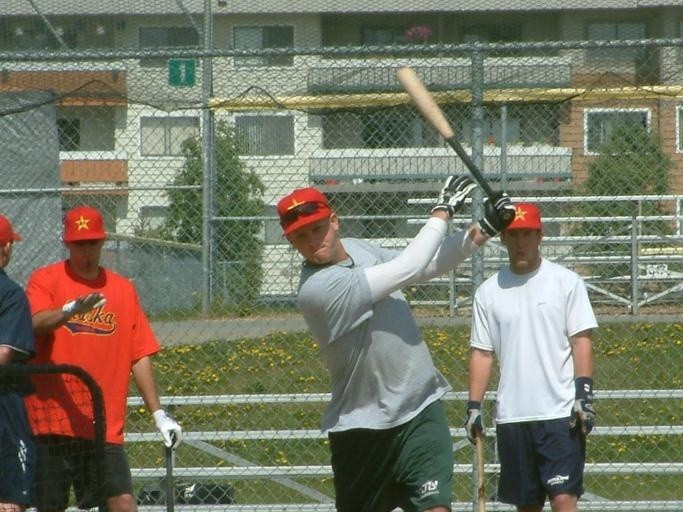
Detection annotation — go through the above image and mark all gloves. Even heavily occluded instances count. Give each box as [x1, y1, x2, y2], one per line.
[151, 408, 183, 450]
[430, 172, 479, 220]
[569, 375, 596, 436]
[62, 291, 107, 319]
[477, 187, 515, 237]
[463, 400, 483, 445]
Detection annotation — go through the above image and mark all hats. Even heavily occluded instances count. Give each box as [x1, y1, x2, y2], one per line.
[63, 206, 106, 242]
[276, 185, 333, 238]
[503, 202, 542, 231]
[0, 214, 23, 246]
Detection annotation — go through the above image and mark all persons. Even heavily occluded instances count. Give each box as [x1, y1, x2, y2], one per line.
[26, 208, 184, 511]
[464, 202, 599, 512]
[277, 175, 516, 512]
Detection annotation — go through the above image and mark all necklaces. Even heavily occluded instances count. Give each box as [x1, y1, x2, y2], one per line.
[303, 252, 355, 268]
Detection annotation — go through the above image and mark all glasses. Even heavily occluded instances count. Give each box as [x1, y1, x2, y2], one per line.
[280, 197, 330, 229]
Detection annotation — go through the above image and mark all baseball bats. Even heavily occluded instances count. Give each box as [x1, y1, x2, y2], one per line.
[393, 64, 498, 204]
